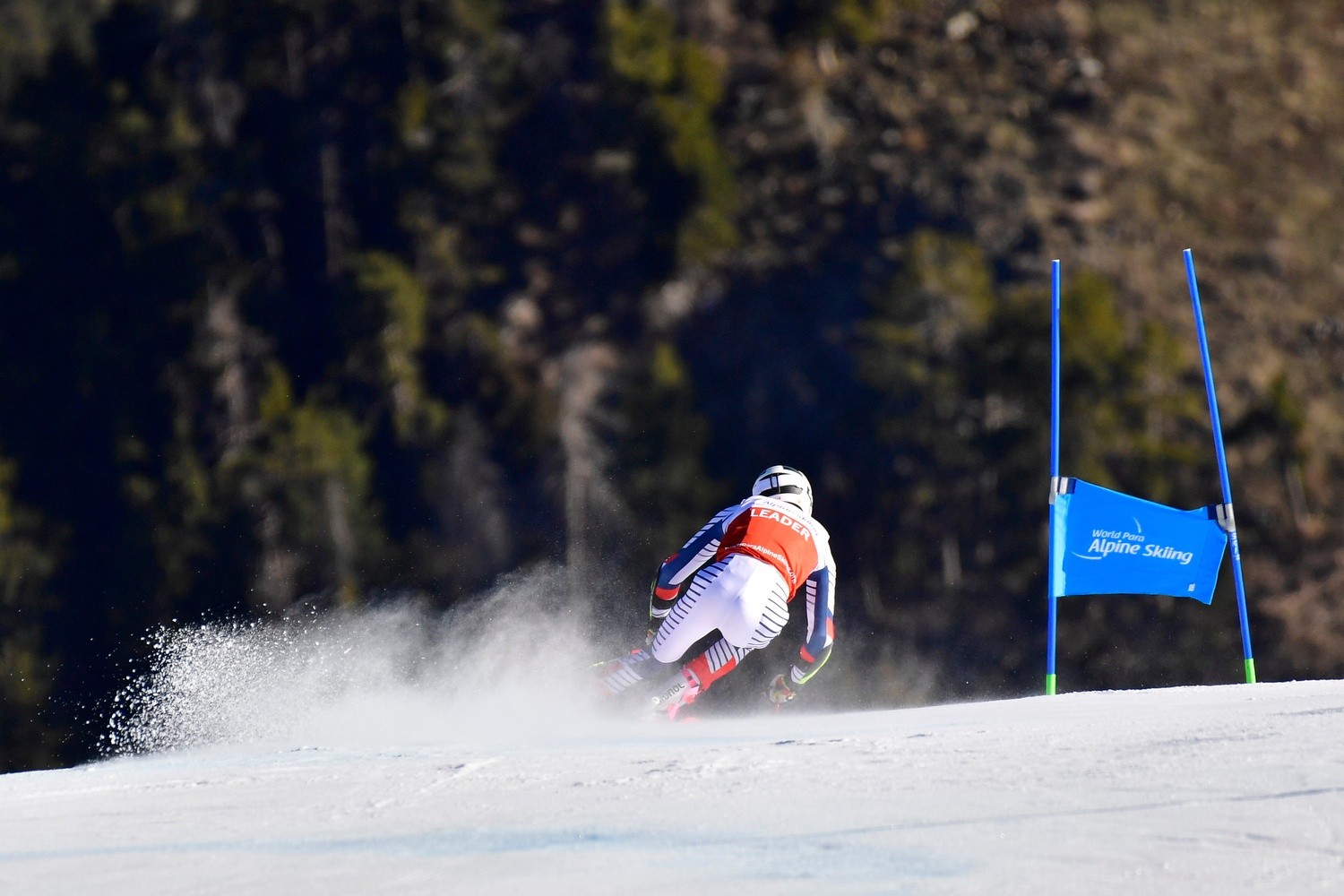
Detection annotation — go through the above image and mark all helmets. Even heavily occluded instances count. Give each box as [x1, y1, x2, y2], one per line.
[752, 464, 812, 517]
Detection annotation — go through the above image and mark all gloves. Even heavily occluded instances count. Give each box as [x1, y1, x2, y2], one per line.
[762, 674, 798, 706]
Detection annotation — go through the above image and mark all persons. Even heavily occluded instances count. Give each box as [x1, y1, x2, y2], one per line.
[574, 466, 836, 745]
